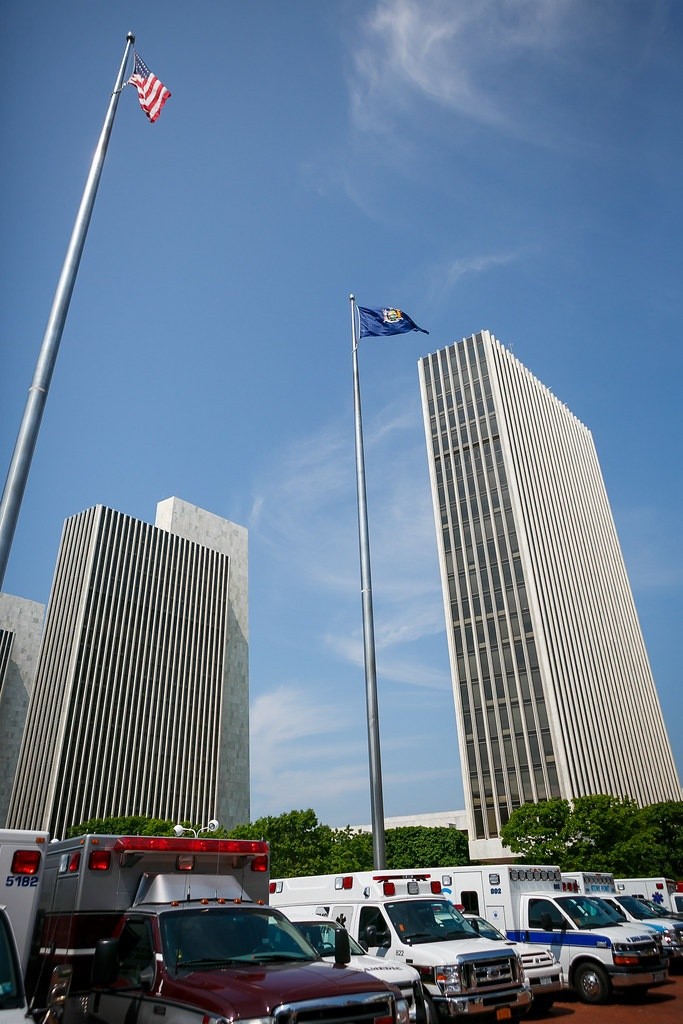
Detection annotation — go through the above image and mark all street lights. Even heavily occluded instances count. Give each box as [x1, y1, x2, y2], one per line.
[174, 820, 220, 838]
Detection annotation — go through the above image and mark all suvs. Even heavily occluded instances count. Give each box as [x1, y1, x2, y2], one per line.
[266, 914, 431, 1024]
[429, 905, 565, 1015]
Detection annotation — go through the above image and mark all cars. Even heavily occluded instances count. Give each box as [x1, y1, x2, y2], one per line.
[635, 898, 683, 921]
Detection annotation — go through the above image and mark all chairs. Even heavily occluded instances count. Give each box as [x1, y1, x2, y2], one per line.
[310, 927, 324, 949]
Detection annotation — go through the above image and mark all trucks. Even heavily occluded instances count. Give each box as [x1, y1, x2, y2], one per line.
[614, 877, 683, 914]
[562, 873, 683, 960]
[388, 865, 669, 1005]
[0, 828, 73, 1024]
[24, 835, 413, 1024]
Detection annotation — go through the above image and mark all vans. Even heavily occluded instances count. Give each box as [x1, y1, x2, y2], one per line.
[268, 871, 534, 1024]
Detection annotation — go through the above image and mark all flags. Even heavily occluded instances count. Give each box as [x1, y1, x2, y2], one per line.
[127, 52, 172, 123]
[355, 305, 429, 340]
